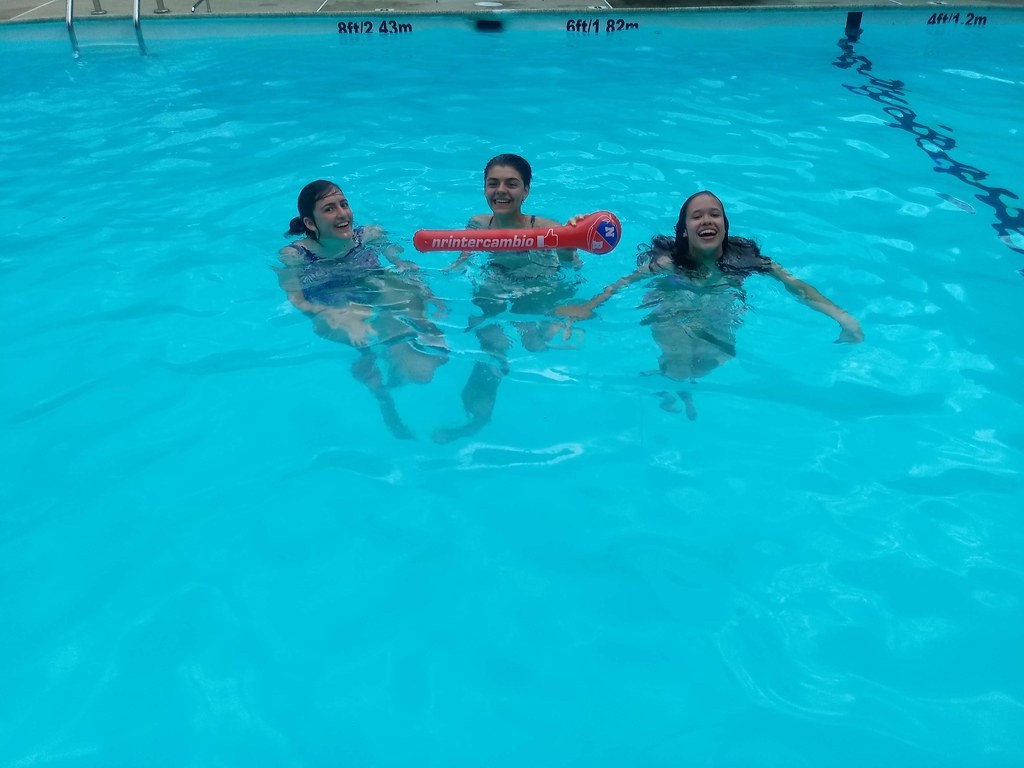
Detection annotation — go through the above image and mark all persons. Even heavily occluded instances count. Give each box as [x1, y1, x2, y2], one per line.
[471, 154, 589, 226]
[656, 190, 729, 261]
[288, 180, 354, 239]
